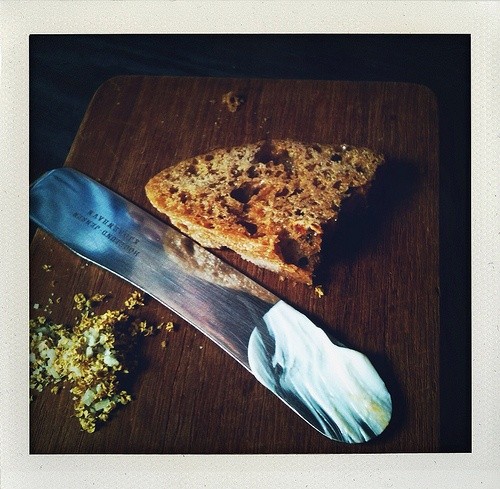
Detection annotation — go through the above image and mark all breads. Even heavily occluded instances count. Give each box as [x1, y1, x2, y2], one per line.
[144, 138, 386, 285]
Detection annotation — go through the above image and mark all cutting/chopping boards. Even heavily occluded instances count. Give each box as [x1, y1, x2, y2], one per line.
[29, 74, 443, 454]
